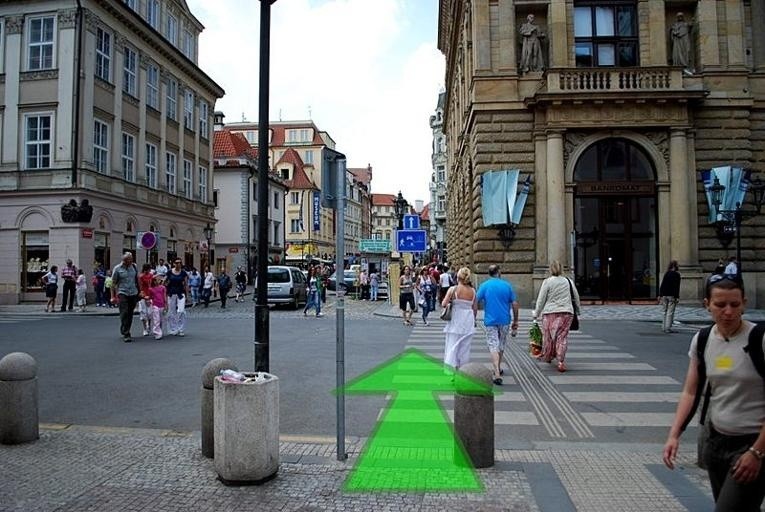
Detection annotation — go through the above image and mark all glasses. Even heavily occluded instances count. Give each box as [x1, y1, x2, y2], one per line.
[706, 273, 742, 286]
[175, 262, 182, 264]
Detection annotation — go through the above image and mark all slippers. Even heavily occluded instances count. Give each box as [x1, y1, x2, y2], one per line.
[494, 377, 503, 385]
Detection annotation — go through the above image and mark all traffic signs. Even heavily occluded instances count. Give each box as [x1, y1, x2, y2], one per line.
[403, 214, 420, 230]
[397, 230, 426, 252]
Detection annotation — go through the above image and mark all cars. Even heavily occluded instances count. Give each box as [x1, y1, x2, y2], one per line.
[251, 256, 360, 311]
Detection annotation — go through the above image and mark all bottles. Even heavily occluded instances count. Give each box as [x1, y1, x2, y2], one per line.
[219, 368, 244, 384]
[511, 328, 517, 338]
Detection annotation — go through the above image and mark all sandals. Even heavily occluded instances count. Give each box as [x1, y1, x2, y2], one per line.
[558, 362, 566, 372]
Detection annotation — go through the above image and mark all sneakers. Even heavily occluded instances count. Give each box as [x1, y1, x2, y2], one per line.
[143, 330, 149, 336]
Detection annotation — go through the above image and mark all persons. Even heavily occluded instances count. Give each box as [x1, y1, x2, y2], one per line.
[662, 272, 764, 512]
[668, 11, 696, 77]
[519, 13, 547, 74]
[41, 251, 738, 386]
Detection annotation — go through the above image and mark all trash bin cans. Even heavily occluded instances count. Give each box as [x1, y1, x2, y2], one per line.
[214, 371, 279, 487]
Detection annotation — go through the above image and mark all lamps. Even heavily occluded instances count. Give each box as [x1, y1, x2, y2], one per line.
[213, 150, 227, 166]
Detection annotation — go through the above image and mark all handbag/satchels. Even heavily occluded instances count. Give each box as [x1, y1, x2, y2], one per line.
[570, 311, 579, 330]
[440, 285, 457, 321]
[448, 274, 455, 286]
[418, 292, 428, 307]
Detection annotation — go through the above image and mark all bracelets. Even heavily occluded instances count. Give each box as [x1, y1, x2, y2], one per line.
[749, 447, 765, 460]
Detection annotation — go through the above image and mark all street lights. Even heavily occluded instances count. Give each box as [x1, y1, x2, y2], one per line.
[391, 191, 409, 259]
[707, 175, 765, 279]
[299, 239, 305, 265]
[203, 222, 213, 272]
